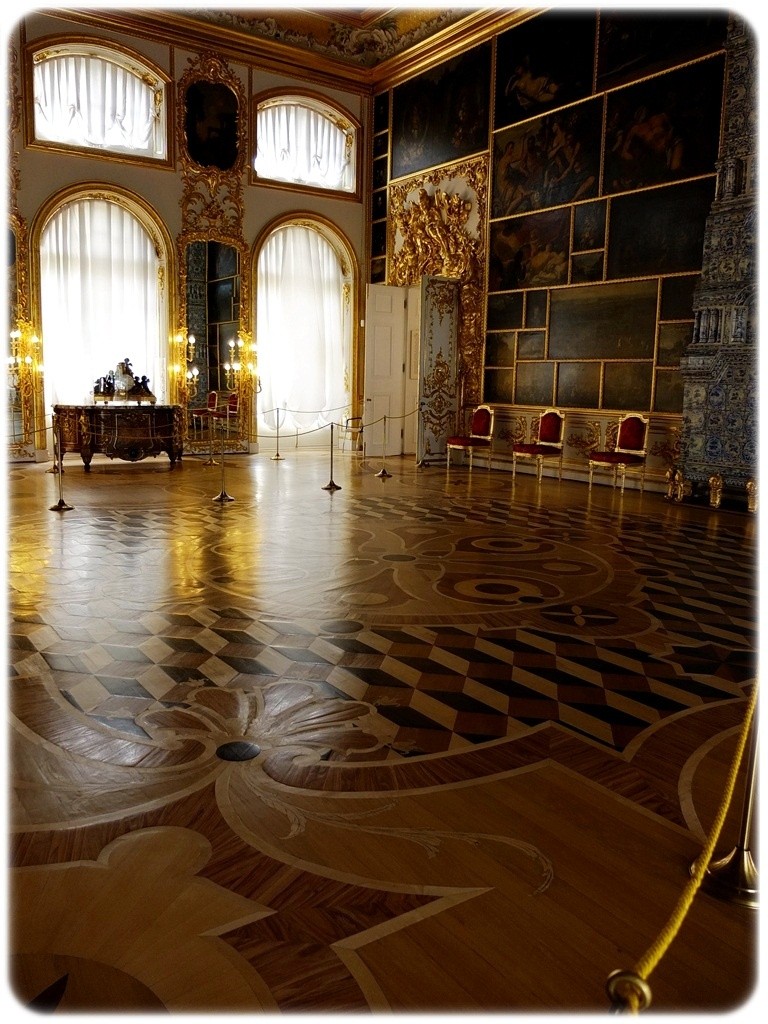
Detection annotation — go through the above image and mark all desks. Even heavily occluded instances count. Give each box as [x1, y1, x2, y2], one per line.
[53, 405, 184, 473]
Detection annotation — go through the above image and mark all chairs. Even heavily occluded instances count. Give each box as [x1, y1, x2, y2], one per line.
[443, 404, 495, 473]
[588, 413, 650, 497]
[512, 409, 565, 485]
[188, 391, 218, 441]
[207, 392, 240, 441]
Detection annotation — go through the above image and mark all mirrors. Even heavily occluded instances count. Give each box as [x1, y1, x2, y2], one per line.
[177, 225, 252, 456]
[173, 49, 248, 197]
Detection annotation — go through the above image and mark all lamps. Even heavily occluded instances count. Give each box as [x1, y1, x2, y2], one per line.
[172, 327, 200, 400]
[222, 327, 262, 400]
[7, 318, 43, 397]
